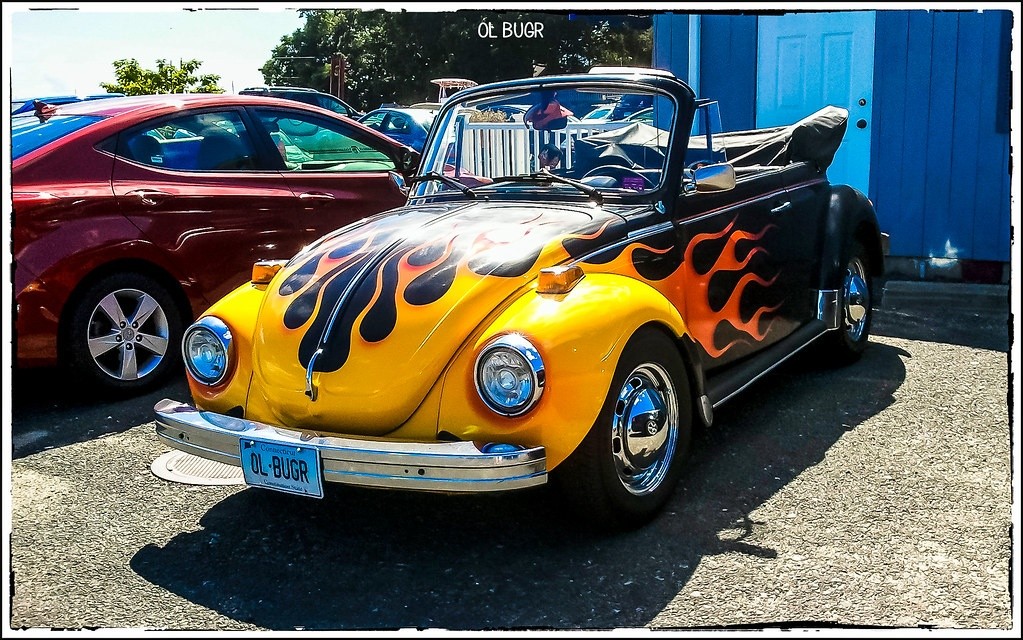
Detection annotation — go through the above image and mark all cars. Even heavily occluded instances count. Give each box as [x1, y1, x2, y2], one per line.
[480, 103, 579, 123]
[10, 94, 494, 398]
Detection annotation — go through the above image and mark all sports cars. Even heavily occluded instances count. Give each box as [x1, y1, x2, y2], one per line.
[151, 72, 884, 516]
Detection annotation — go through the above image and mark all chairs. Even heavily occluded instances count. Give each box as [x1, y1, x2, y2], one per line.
[133, 134, 170, 169]
[198, 125, 249, 170]
